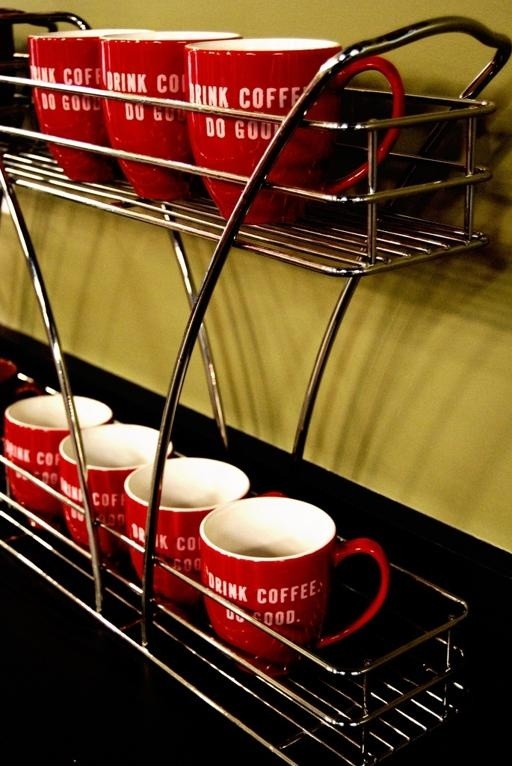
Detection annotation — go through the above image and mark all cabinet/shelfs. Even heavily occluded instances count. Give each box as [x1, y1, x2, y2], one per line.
[0, 9, 512, 766]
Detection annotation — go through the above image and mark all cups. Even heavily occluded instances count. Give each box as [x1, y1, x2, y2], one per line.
[55, 421, 175, 556]
[121, 456, 264, 624]
[5, 392, 113, 514]
[195, 493, 389, 672]
[183, 36, 403, 226]
[23, 28, 239, 203]
